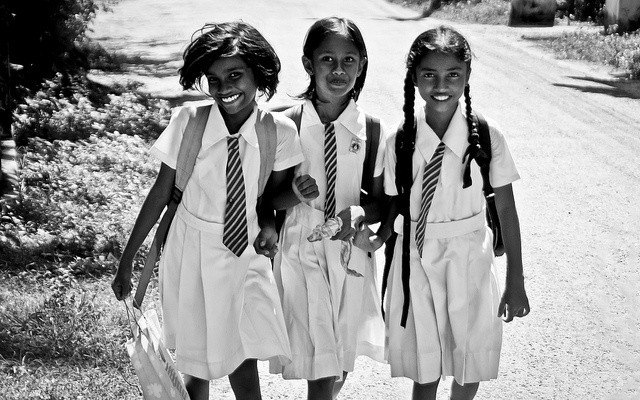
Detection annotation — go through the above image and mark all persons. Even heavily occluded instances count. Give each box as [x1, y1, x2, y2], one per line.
[352, 26, 531, 400]
[252, 17, 387, 399]
[111, 18, 305, 400]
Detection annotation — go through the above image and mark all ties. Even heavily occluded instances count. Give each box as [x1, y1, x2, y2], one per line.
[414, 142, 446, 259]
[324, 123, 336, 223]
[222, 136, 249, 258]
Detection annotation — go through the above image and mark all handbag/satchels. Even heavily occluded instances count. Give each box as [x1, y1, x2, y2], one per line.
[114, 287, 192, 400]
